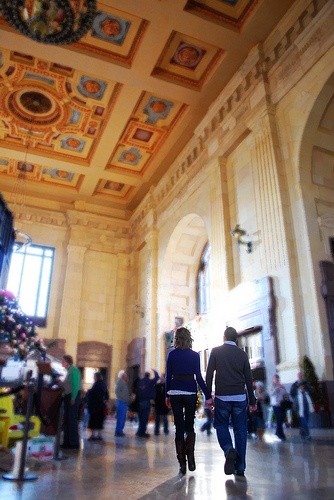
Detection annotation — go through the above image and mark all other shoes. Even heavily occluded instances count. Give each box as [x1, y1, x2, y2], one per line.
[180, 456, 187, 474]
[188, 454, 196, 471]
[224, 448, 239, 475]
[236, 472, 244, 476]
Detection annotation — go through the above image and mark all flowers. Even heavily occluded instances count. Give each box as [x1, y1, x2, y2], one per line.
[0, 288, 48, 363]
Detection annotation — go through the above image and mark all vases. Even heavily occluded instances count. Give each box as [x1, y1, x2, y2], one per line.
[0, 341, 12, 362]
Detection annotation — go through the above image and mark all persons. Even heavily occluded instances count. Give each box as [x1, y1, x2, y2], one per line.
[84, 371, 110, 440]
[201, 393, 215, 435]
[205, 326, 258, 476]
[244, 370, 318, 443]
[57, 354, 82, 450]
[114, 367, 170, 437]
[164, 327, 213, 472]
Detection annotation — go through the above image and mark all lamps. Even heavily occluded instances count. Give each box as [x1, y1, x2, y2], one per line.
[11, 102, 42, 251]
[232, 225, 253, 254]
